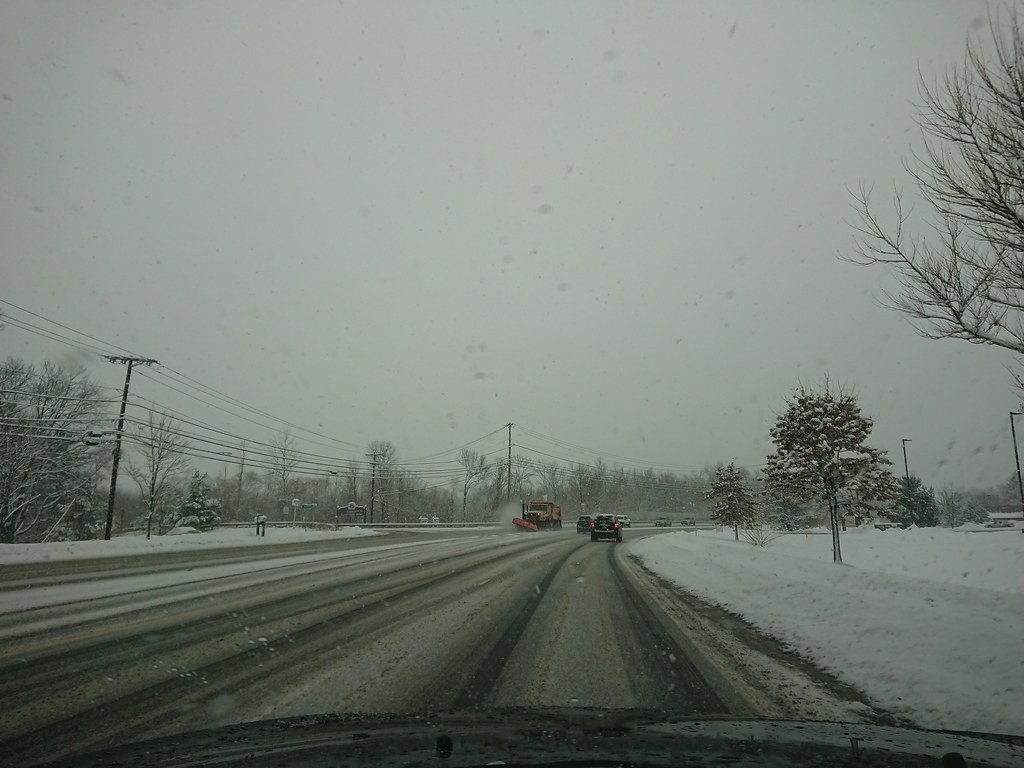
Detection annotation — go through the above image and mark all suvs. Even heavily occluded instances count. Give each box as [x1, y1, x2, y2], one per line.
[576, 514, 593, 531]
[655, 515, 673, 526]
[591, 514, 623, 544]
[681, 516, 697, 526]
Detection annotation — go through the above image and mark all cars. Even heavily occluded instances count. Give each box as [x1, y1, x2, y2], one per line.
[616, 514, 631, 528]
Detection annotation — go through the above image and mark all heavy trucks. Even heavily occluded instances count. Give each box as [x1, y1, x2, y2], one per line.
[522, 501, 562, 528]
